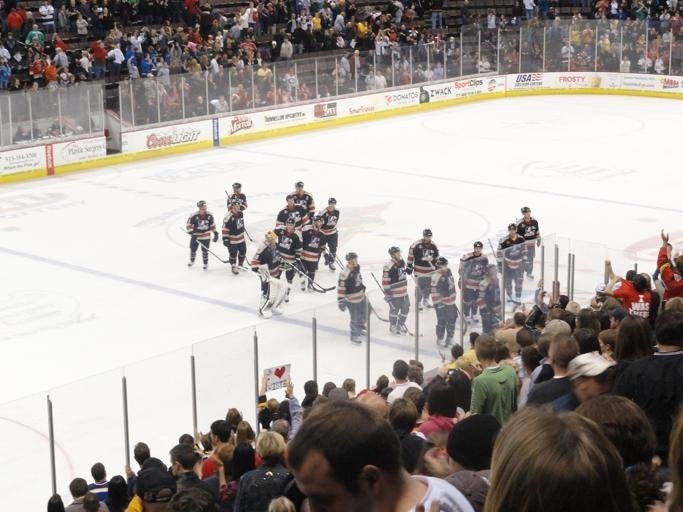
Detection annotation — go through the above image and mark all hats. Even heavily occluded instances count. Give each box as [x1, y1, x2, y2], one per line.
[446, 412, 500, 508]
[135, 467, 177, 503]
[567, 350, 616, 382]
[606, 308, 629, 321]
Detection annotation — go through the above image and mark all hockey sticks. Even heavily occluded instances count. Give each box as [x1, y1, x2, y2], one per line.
[279, 256, 336, 291]
[370, 272, 424, 337]
[487, 239, 520, 304]
[427, 258, 468, 336]
[224, 189, 262, 243]
[186, 231, 230, 264]
[295, 258, 325, 293]
[364, 294, 391, 322]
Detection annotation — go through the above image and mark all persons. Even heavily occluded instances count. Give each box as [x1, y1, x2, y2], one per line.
[337, 227, 502, 349]
[516, 207, 541, 279]
[496, 223, 530, 305]
[165, 330, 522, 512]
[253, 181, 339, 319]
[47, 440, 175, 511]
[222, 202, 247, 274]
[186, 200, 218, 269]
[0, 1, 683, 143]
[486, 230, 683, 510]
[227, 183, 248, 213]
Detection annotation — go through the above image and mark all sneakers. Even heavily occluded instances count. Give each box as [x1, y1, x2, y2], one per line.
[259, 282, 523, 348]
